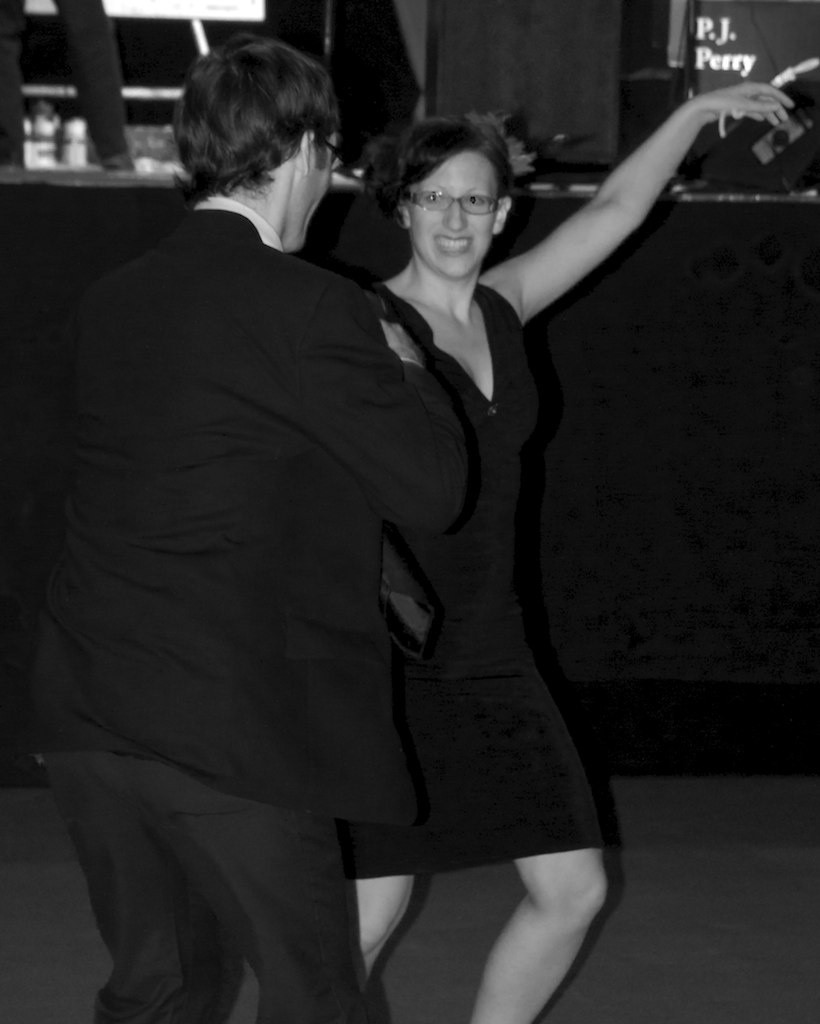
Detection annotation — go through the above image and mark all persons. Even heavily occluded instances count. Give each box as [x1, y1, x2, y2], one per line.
[0, 33, 470, 1024]
[336, 81, 794, 1024]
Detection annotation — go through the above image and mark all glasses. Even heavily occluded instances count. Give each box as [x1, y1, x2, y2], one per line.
[402, 189, 498, 215]
[320, 136, 346, 170]
